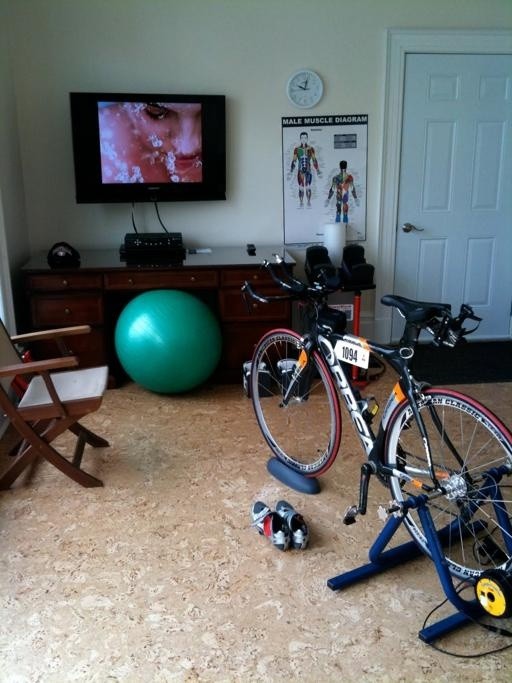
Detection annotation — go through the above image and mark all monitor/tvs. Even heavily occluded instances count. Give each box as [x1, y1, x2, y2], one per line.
[69, 90, 226, 203]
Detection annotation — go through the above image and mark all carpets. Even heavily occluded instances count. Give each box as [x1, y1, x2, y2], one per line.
[385, 339, 512, 387]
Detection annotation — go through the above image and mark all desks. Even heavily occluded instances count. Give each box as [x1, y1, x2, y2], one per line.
[21, 243, 297, 388]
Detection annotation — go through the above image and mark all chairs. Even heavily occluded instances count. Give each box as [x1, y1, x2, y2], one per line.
[1, 318, 110, 490]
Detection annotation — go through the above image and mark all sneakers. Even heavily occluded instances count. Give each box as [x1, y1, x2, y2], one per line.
[249, 501, 292, 552]
[274, 500, 311, 550]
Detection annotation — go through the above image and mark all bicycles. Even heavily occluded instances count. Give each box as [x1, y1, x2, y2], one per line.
[241, 255, 512, 585]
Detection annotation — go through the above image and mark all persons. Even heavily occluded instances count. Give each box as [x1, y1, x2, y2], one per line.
[98, 98, 202, 183]
[286, 131, 322, 210]
[324, 159, 359, 226]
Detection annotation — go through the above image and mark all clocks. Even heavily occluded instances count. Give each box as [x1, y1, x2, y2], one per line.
[286, 70, 323, 108]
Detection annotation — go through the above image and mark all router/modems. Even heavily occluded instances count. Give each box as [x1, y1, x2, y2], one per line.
[120, 233, 186, 254]
[120, 253, 186, 267]
